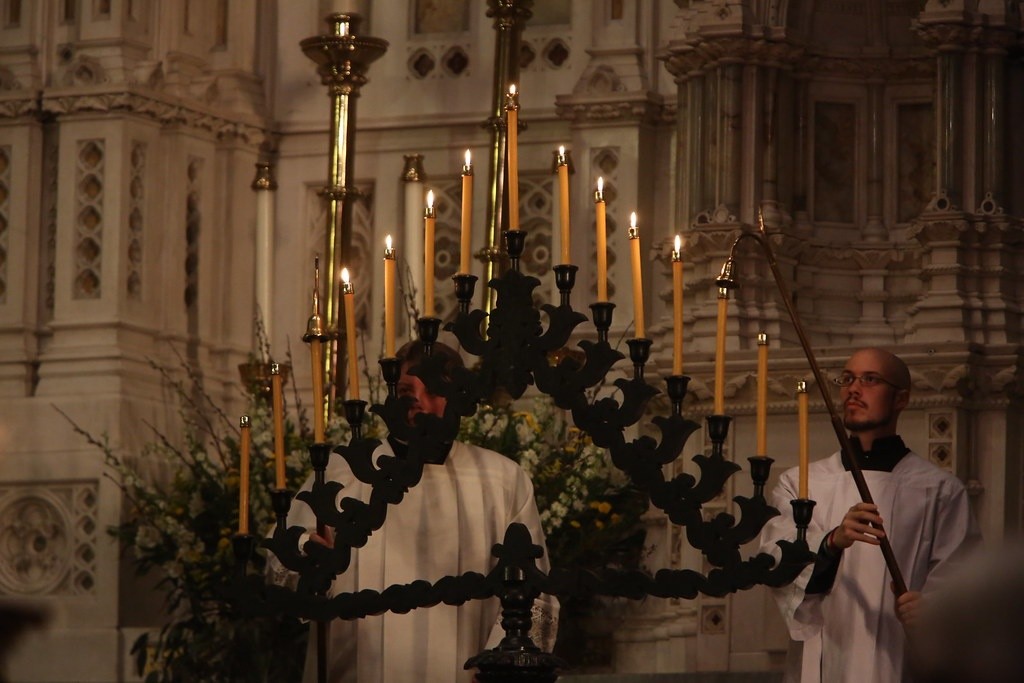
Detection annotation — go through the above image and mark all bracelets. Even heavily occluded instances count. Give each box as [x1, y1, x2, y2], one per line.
[830, 525, 844, 551]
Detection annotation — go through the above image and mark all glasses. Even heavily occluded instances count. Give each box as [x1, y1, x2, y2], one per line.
[833, 373, 901, 389]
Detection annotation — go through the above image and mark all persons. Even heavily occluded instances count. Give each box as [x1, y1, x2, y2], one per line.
[267, 342, 561, 683]
[757, 348, 986, 683]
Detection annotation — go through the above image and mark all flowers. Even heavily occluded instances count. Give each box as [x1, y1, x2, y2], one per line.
[48, 261, 658, 683]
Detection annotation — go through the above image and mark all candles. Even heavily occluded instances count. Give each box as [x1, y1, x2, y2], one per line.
[239, 0, 809, 537]
